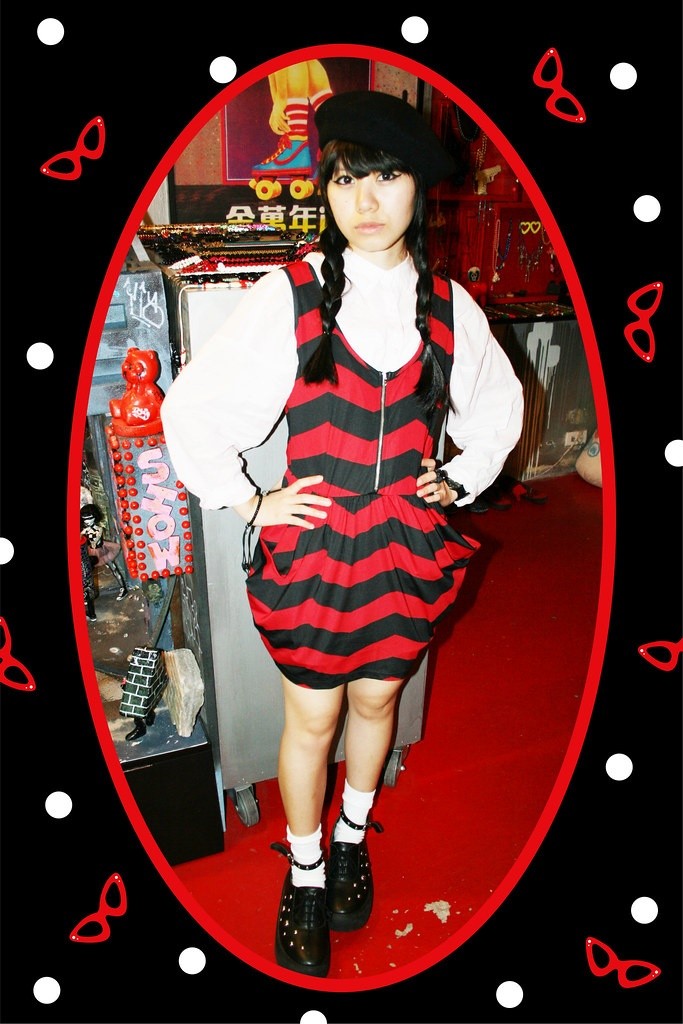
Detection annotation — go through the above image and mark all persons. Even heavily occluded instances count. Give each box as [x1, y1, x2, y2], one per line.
[80, 504, 129, 601]
[160, 90, 524, 978]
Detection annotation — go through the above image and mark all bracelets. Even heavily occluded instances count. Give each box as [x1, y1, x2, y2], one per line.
[241, 494, 263, 574]
[441, 470, 467, 501]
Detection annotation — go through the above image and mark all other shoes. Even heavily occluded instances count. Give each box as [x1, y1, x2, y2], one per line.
[466, 495, 487, 513]
[506, 476, 548, 502]
[487, 487, 512, 510]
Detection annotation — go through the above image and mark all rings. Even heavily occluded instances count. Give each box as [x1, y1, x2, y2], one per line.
[429, 470, 442, 483]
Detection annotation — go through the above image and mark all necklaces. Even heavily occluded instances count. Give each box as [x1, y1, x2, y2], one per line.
[428, 94, 575, 320]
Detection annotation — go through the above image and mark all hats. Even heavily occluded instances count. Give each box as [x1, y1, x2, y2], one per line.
[315, 90, 454, 188]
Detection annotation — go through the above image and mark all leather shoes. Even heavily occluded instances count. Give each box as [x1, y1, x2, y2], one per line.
[325, 804, 382, 930]
[270, 842, 330, 977]
